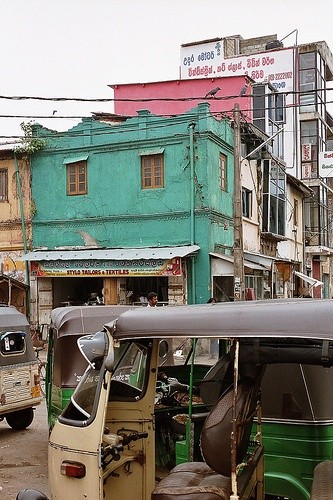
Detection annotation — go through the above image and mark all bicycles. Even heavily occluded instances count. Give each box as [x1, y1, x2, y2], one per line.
[34, 348, 47, 397]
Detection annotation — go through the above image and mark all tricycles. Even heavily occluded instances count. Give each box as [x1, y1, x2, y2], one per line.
[0, 306, 47, 429]
[16, 298, 332, 499]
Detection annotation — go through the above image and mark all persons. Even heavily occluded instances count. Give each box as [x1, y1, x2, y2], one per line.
[207, 298, 216, 304]
[89, 287, 105, 302]
[146, 292, 158, 307]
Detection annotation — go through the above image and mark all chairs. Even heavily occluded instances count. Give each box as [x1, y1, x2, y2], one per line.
[151, 353, 258, 500]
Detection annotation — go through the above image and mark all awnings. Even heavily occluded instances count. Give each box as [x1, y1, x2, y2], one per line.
[14, 245, 201, 262]
[294, 271, 325, 288]
